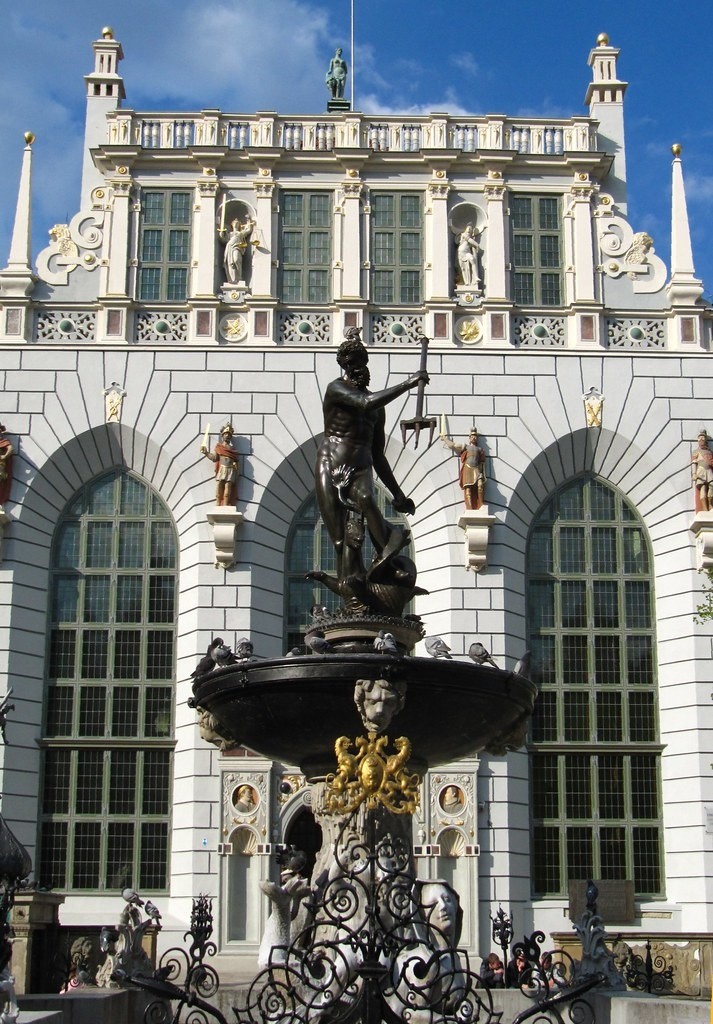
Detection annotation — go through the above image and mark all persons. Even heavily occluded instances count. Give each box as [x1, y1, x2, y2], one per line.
[69, 936, 95, 984]
[353, 679, 407, 732]
[441, 786, 462, 814]
[416, 881, 458, 951]
[476, 951, 565, 989]
[199, 426, 238, 508]
[233, 785, 255, 813]
[218, 215, 254, 285]
[691, 429, 713, 512]
[315, 339, 429, 579]
[457, 221, 484, 286]
[440, 431, 489, 510]
[325, 48, 348, 97]
[0, 423, 14, 505]
